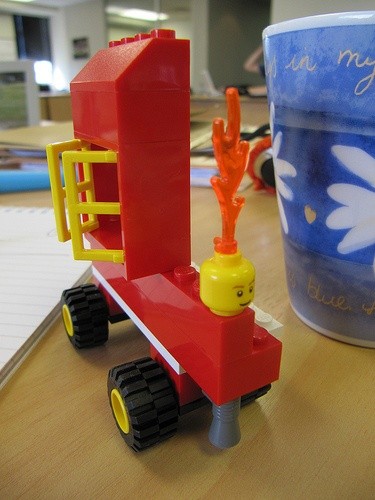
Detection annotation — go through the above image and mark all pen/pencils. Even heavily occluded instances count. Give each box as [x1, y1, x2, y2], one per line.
[245, 122, 270, 142]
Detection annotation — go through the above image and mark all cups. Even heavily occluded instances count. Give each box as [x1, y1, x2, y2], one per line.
[263, 11, 375, 351]
